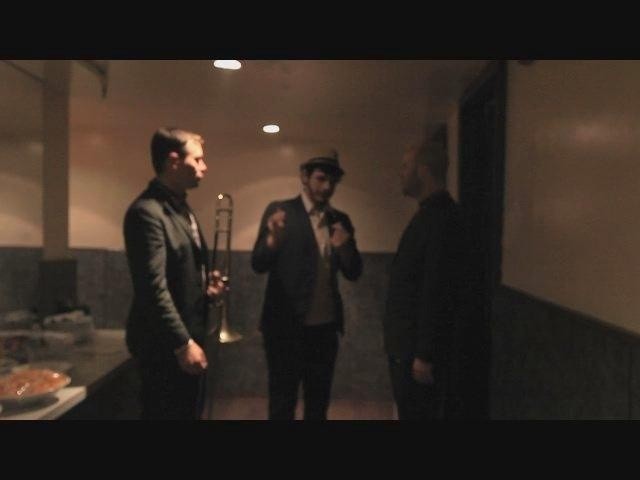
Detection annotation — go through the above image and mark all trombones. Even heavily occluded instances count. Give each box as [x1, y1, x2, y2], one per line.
[196, 194, 244, 424]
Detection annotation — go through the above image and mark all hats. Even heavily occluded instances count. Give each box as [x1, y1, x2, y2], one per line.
[300, 151, 345, 176]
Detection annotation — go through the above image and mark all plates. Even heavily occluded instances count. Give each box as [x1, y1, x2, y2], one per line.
[0, 369, 73, 410]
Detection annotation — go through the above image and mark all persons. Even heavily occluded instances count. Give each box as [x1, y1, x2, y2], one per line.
[382, 141, 473, 420]
[122, 126, 230, 420]
[250, 146, 362, 421]
